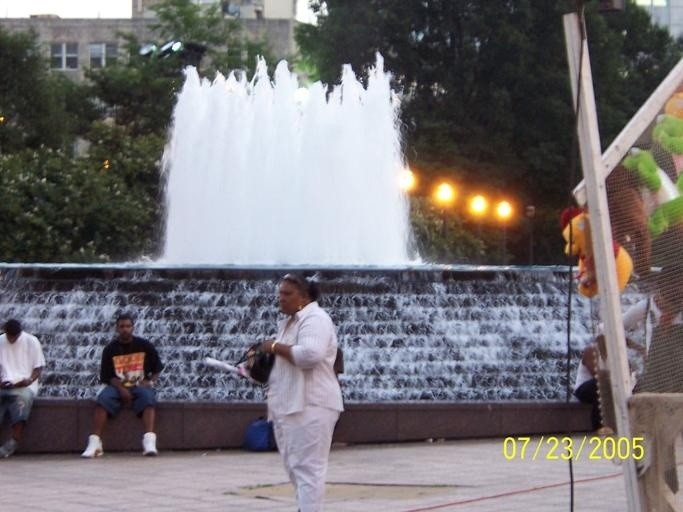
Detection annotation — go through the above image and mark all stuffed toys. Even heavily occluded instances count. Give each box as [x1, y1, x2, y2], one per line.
[561, 89, 683, 297]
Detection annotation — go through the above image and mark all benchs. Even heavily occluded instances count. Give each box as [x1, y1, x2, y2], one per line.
[0, 397, 596, 454]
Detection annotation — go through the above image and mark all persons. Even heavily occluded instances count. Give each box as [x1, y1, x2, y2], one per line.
[621, 265, 683, 494]
[248, 274, 344, 512]
[0, 319, 46, 459]
[80, 315, 165, 458]
[573, 323, 638, 436]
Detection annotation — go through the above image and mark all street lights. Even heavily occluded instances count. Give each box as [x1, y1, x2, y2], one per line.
[469, 194, 487, 266]
[130, 38, 182, 142]
[525, 203, 538, 265]
[435, 182, 452, 264]
[496, 200, 514, 264]
[399, 169, 417, 262]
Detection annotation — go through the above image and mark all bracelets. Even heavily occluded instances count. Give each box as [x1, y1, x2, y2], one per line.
[271, 342, 277, 354]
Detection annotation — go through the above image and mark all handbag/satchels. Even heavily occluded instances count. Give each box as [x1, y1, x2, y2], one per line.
[245, 419, 275, 451]
[234, 341, 274, 383]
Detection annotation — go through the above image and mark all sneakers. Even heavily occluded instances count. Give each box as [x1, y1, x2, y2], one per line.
[0, 437, 20, 458]
[81, 434, 104, 457]
[142, 432, 158, 455]
